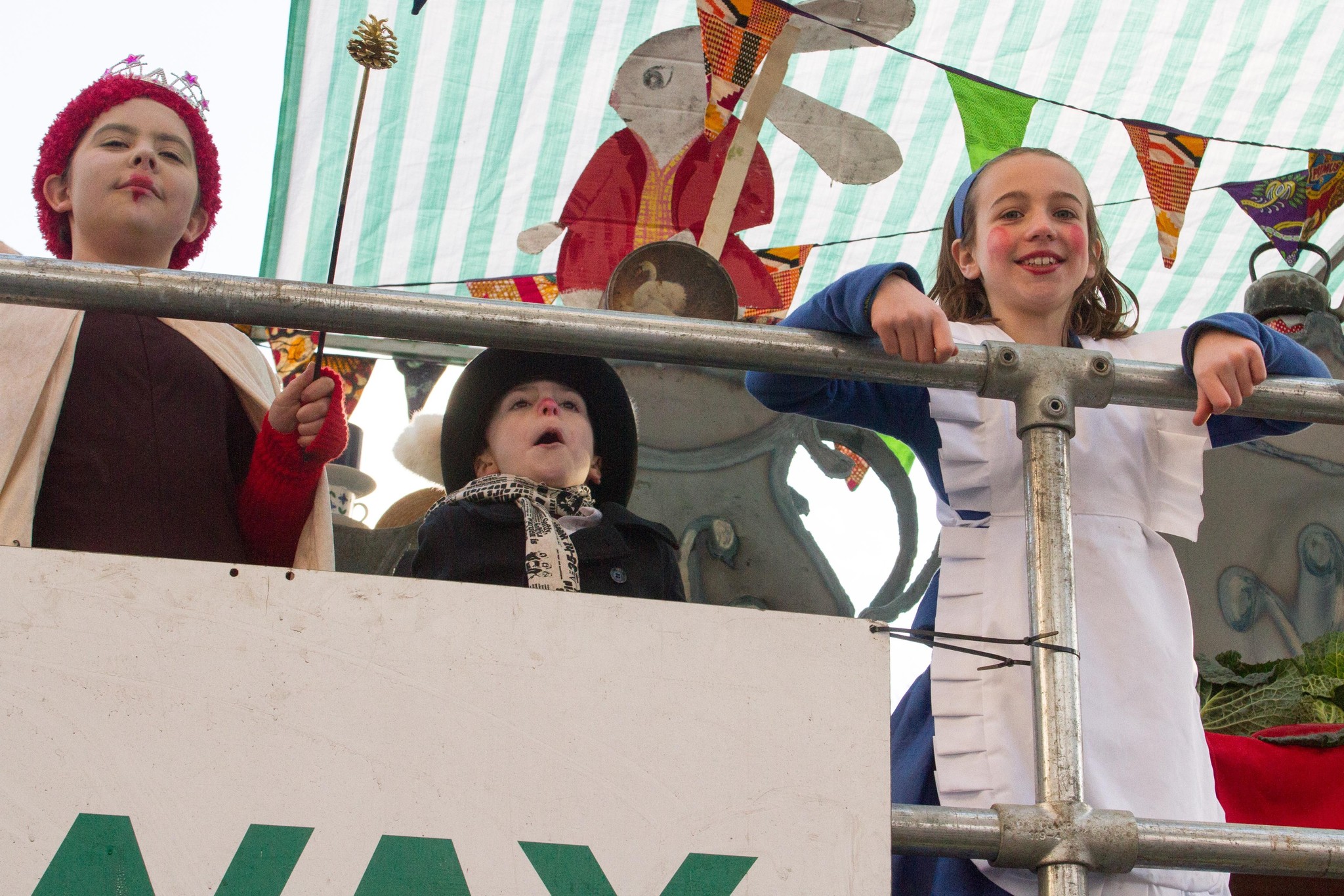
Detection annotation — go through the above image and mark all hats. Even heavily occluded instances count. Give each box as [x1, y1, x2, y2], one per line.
[32, 55, 221, 269]
[439, 350, 638, 511]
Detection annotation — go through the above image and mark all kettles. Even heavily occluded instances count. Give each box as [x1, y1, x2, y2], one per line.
[1243, 242, 1344, 324]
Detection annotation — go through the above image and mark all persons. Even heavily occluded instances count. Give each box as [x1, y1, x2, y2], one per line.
[411, 344, 686, 603]
[741, 148, 1333, 896]
[1, 75, 349, 568]
